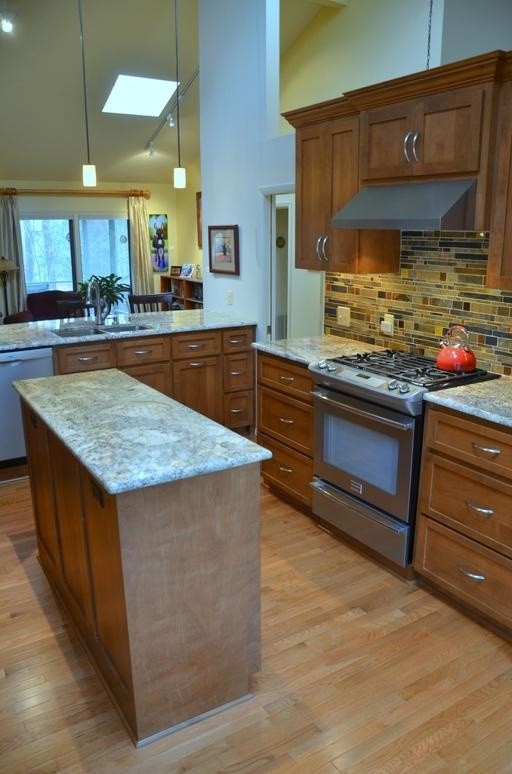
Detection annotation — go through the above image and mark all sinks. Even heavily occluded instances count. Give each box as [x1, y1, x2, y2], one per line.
[51, 326, 102, 337]
[100, 324, 153, 332]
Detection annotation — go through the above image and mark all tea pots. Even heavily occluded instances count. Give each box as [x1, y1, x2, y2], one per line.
[436, 325, 475, 372]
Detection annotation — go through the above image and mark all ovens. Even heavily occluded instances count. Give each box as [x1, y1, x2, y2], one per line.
[309, 386, 416, 571]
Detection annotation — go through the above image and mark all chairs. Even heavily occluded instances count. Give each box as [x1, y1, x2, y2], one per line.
[56, 298, 104, 318]
[128, 293, 173, 312]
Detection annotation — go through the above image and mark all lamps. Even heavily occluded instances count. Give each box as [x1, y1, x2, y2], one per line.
[0, 10, 18, 35]
[76, 1, 99, 189]
[0, 255, 20, 317]
[171, 1, 188, 188]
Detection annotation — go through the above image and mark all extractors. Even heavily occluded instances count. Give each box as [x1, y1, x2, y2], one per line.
[332, 179, 476, 231]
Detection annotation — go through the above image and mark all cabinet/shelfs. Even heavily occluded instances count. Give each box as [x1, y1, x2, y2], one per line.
[410, 409, 512, 639]
[55, 331, 255, 429]
[160, 275, 203, 311]
[253, 355, 316, 513]
[278, 49, 512, 288]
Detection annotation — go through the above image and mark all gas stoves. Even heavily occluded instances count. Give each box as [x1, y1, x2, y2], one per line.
[308, 350, 501, 417]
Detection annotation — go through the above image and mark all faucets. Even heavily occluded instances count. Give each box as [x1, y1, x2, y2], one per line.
[86, 279, 101, 325]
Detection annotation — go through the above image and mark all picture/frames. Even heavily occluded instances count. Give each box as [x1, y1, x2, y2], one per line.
[169, 264, 181, 276]
[207, 224, 240, 276]
